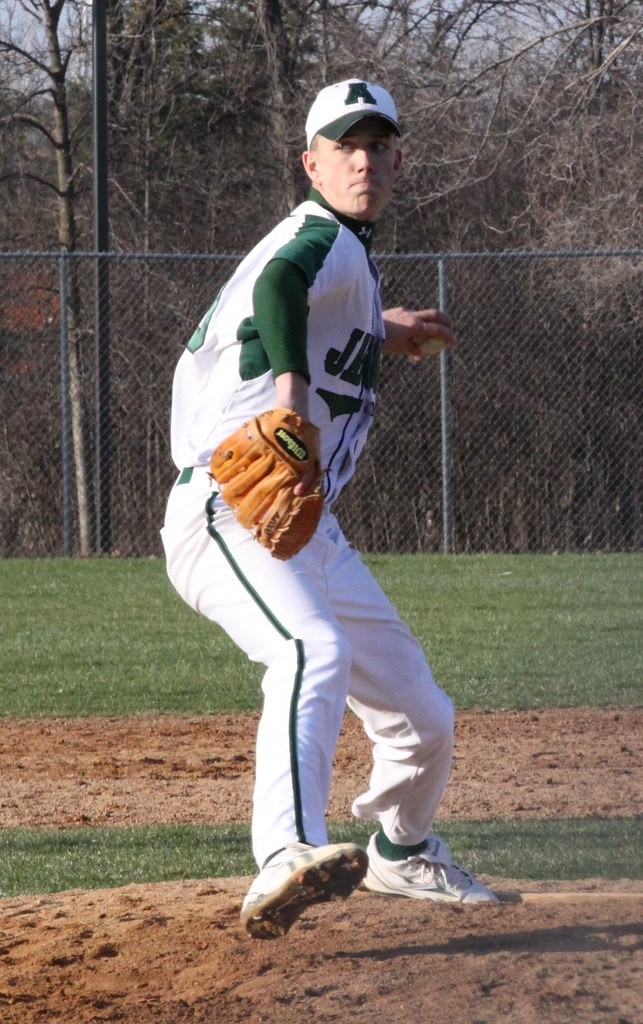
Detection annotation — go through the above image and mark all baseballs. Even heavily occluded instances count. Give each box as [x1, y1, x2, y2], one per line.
[417, 337, 447, 357]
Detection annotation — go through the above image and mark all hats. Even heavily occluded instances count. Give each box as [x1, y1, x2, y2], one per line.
[305, 78, 403, 152]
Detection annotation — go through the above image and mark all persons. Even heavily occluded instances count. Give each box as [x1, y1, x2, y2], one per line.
[159, 80, 500, 939]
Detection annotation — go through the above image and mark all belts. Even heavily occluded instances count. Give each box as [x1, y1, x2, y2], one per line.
[172, 466, 194, 484]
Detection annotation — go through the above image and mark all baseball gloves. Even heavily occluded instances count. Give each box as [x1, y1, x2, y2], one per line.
[206, 406, 328, 562]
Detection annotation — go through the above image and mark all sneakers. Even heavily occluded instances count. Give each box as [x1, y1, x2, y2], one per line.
[362, 831, 501, 907]
[240, 841, 369, 941]
